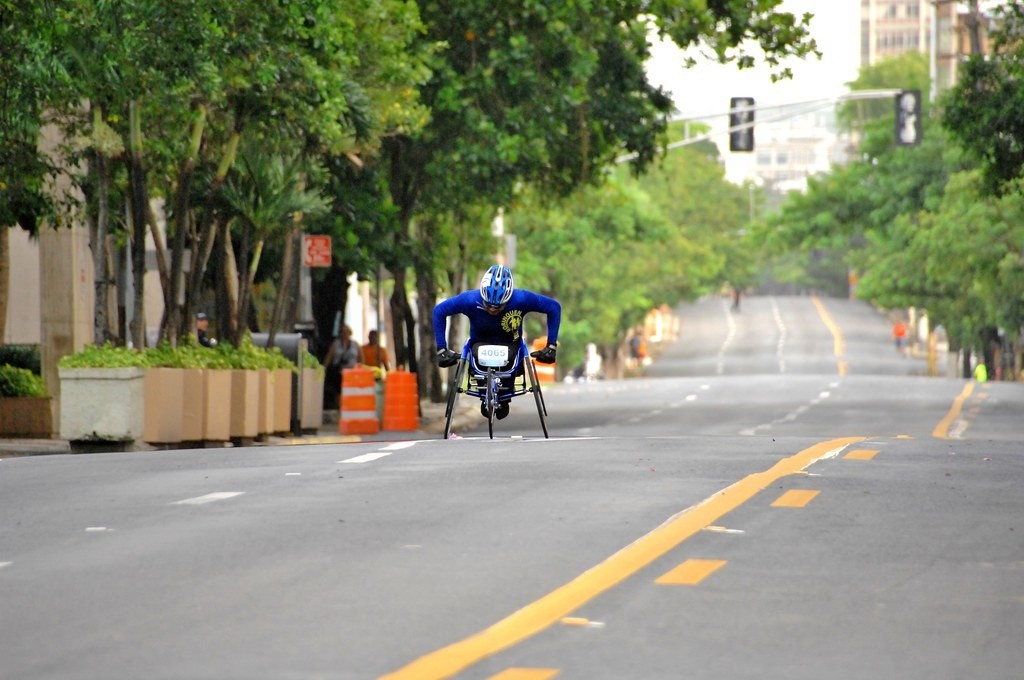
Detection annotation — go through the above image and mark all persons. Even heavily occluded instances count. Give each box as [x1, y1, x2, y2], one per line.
[891, 317, 910, 353]
[322, 324, 365, 411]
[432, 264, 562, 420]
[361, 330, 390, 382]
[197, 312, 213, 349]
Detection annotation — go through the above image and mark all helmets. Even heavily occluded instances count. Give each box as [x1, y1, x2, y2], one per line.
[480, 265, 513, 304]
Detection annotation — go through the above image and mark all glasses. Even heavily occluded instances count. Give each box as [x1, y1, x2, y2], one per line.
[485, 301, 502, 309]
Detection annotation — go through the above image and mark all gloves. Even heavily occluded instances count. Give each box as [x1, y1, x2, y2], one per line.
[530, 342, 557, 364]
[437, 349, 461, 368]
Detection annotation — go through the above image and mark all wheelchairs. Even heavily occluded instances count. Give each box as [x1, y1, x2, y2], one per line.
[442, 338, 549, 439]
